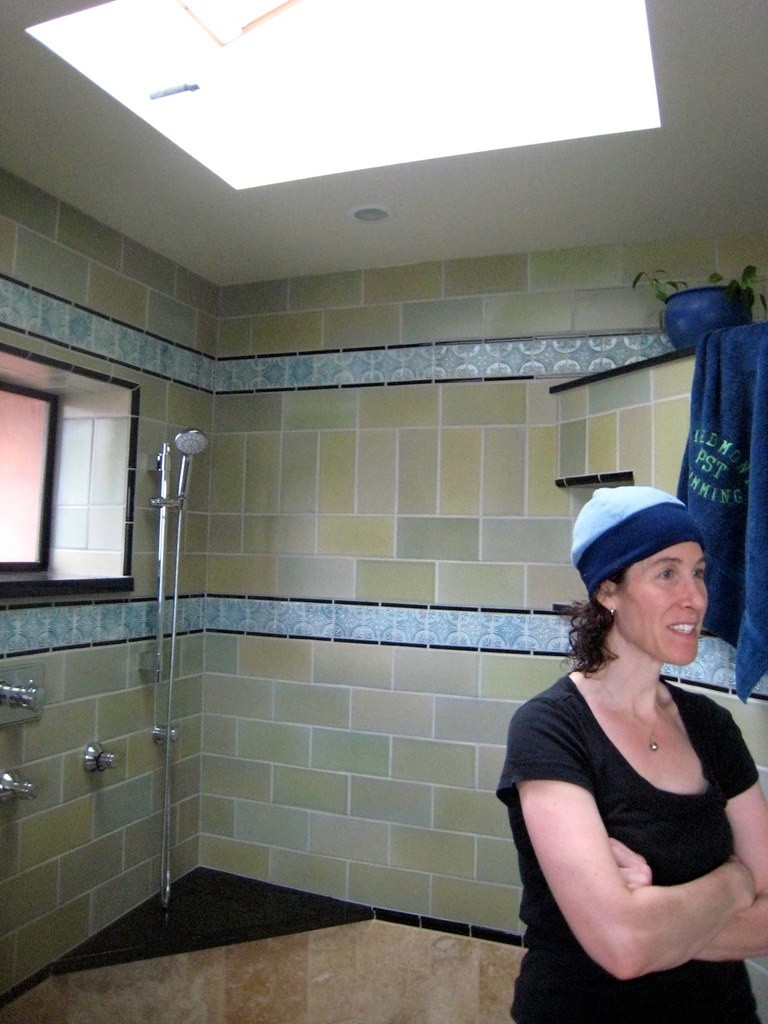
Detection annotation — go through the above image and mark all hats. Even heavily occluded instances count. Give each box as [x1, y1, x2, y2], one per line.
[569, 486, 705, 605]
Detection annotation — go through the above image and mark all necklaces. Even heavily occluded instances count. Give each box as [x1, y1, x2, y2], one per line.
[599, 678, 658, 750]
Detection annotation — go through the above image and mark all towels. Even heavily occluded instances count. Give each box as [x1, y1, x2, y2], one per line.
[677, 321, 768, 704]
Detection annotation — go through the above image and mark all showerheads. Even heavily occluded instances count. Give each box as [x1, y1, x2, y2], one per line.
[175, 429, 209, 458]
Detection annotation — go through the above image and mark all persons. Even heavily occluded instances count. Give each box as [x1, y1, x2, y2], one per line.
[496, 487, 768, 1024]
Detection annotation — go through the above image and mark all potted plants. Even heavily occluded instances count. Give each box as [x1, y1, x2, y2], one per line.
[631, 263, 768, 352]
